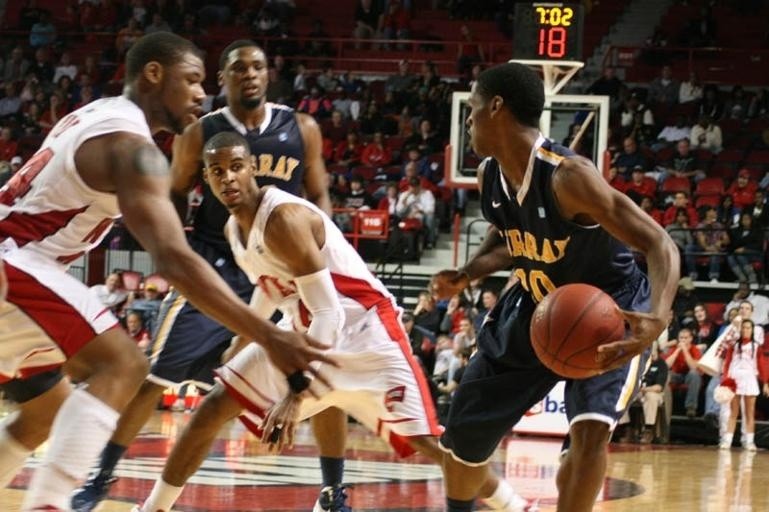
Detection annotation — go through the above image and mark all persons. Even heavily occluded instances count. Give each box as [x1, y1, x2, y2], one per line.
[1, 1, 768, 512]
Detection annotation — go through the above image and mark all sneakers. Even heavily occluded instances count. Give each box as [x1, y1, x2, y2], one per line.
[311, 482, 352, 512]
[740, 433, 758, 451]
[718, 433, 734, 449]
[70, 470, 120, 511]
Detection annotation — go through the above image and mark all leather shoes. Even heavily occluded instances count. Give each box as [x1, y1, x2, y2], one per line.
[620, 431, 633, 444]
[640, 431, 655, 444]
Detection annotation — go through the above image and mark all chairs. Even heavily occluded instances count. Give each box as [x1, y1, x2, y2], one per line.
[4, 0, 483, 356]
[406, 1, 769, 411]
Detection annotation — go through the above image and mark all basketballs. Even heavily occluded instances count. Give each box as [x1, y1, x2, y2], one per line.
[530, 283, 624, 379]
